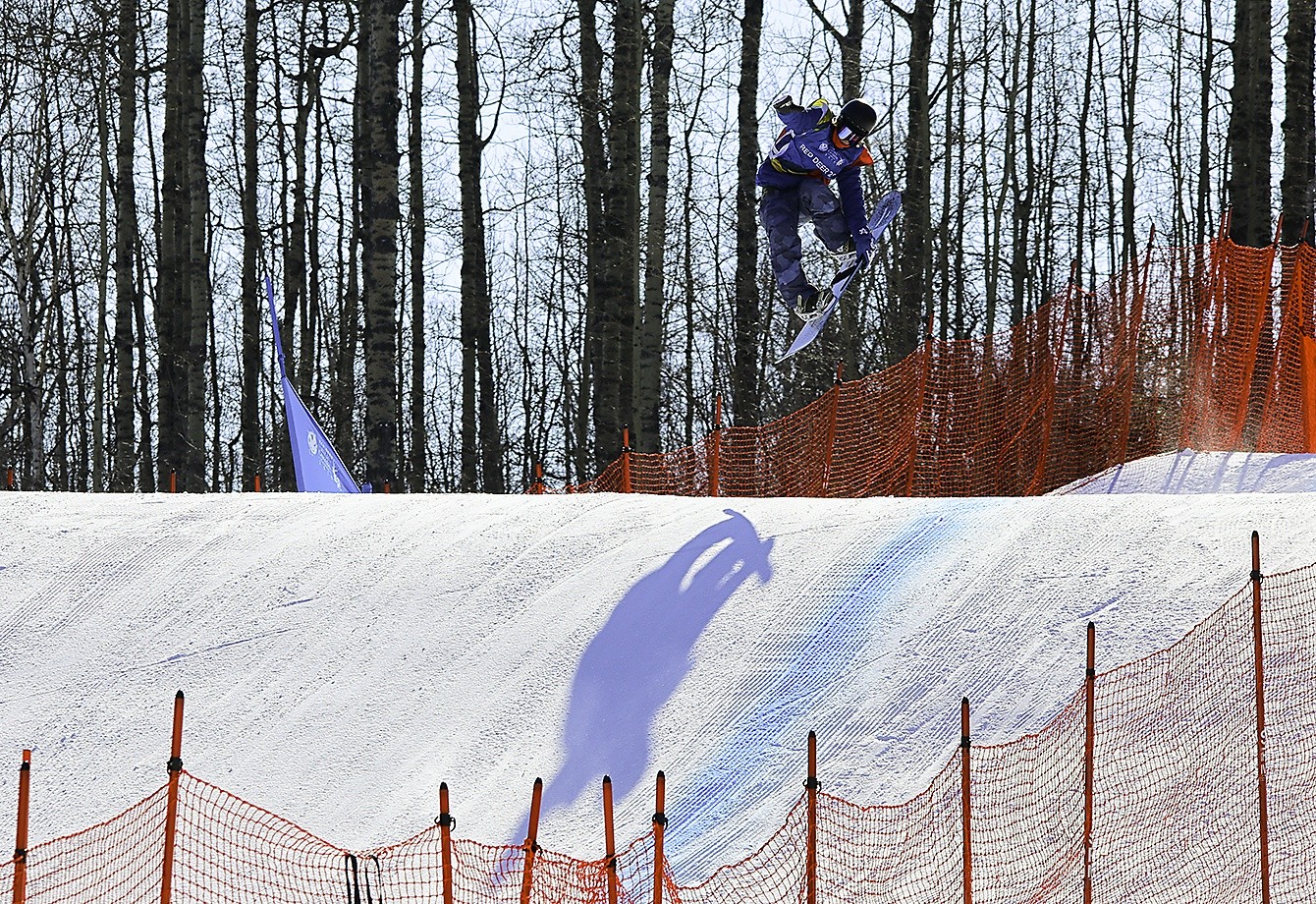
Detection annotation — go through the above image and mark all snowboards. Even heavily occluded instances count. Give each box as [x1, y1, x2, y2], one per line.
[772, 190, 903, 365]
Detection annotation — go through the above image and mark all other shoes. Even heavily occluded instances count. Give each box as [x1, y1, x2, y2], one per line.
[830, 238, 859, 258]
[794, 288, 832, 321]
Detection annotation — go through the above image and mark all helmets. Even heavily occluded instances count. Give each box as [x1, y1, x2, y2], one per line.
[841, 99, 876, 134]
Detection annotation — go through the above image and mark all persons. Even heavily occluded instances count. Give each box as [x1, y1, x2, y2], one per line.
[756, 94, 877, 315]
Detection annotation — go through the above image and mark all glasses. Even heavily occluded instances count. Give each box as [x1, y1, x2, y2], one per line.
[837, 125, 866, 147]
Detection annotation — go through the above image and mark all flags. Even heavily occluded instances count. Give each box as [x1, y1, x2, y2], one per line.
[284, 378, 362, 494]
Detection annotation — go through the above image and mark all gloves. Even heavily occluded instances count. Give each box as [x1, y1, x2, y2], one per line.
[774, 96, 805, 114]
[855, 237, 876, 264]
